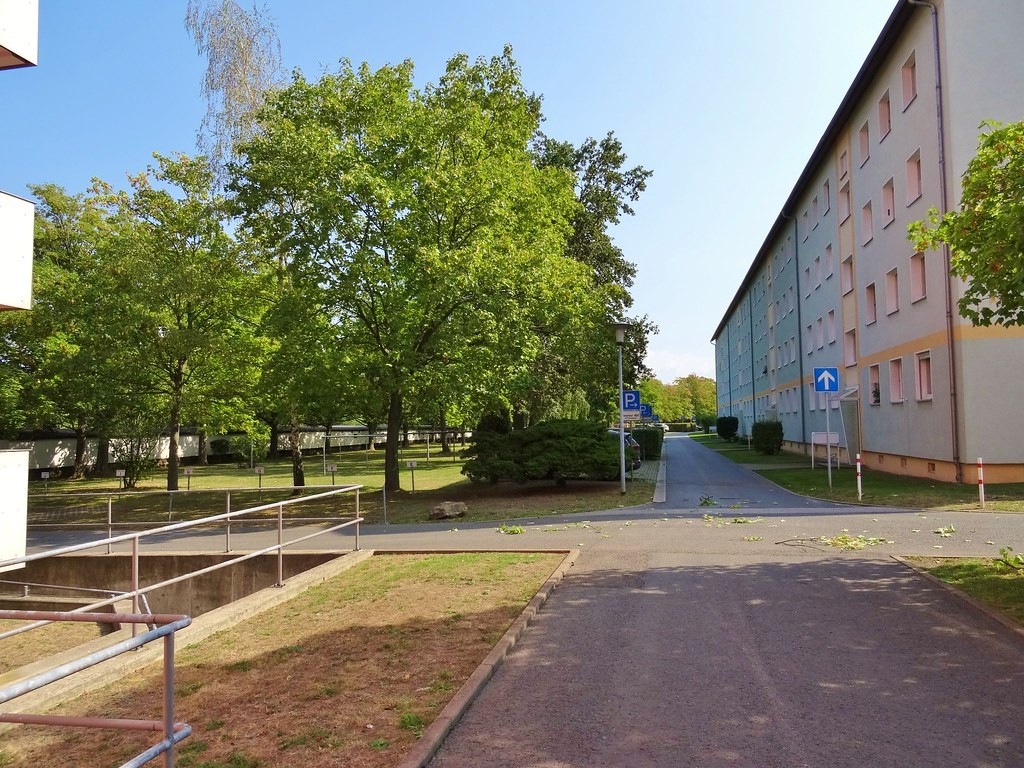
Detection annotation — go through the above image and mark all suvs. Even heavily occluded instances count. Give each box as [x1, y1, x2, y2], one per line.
[604, 429, 641, 481]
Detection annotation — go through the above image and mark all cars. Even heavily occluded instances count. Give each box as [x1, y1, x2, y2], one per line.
[648, 423, 670, 432]
[695, 426, 704, 430]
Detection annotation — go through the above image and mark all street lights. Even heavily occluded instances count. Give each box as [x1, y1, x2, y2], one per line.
[651, 402, 656, 421]
[606, 322, 635, 495]
